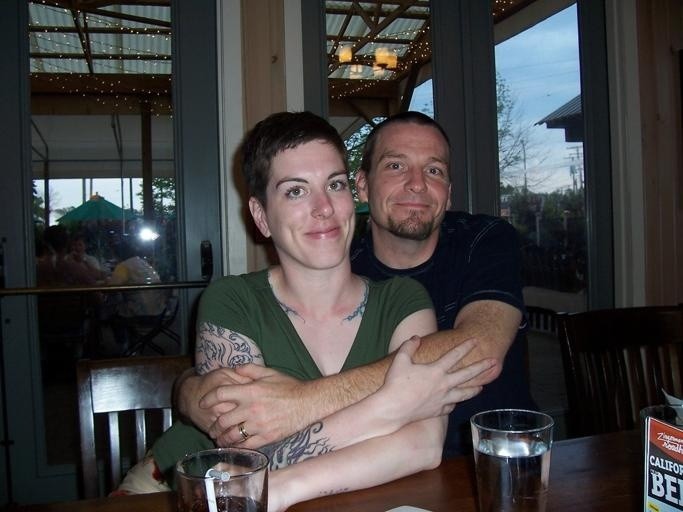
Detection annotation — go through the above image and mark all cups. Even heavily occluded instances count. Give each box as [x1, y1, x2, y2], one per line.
[174, 447, 269, 511]
[470, 408, 555, 511]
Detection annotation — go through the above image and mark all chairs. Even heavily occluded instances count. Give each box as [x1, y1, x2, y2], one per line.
[76, 354, 195, 499]
[514, 305, 572, 415]
[557, 306, 683, 440]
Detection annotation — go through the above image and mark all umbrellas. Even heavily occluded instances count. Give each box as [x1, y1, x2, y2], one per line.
[55, 194, 139, 225]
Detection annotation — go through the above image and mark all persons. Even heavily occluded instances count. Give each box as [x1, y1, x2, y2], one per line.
[106, 111, 541, 511]
[34, 234, 167, 358]
